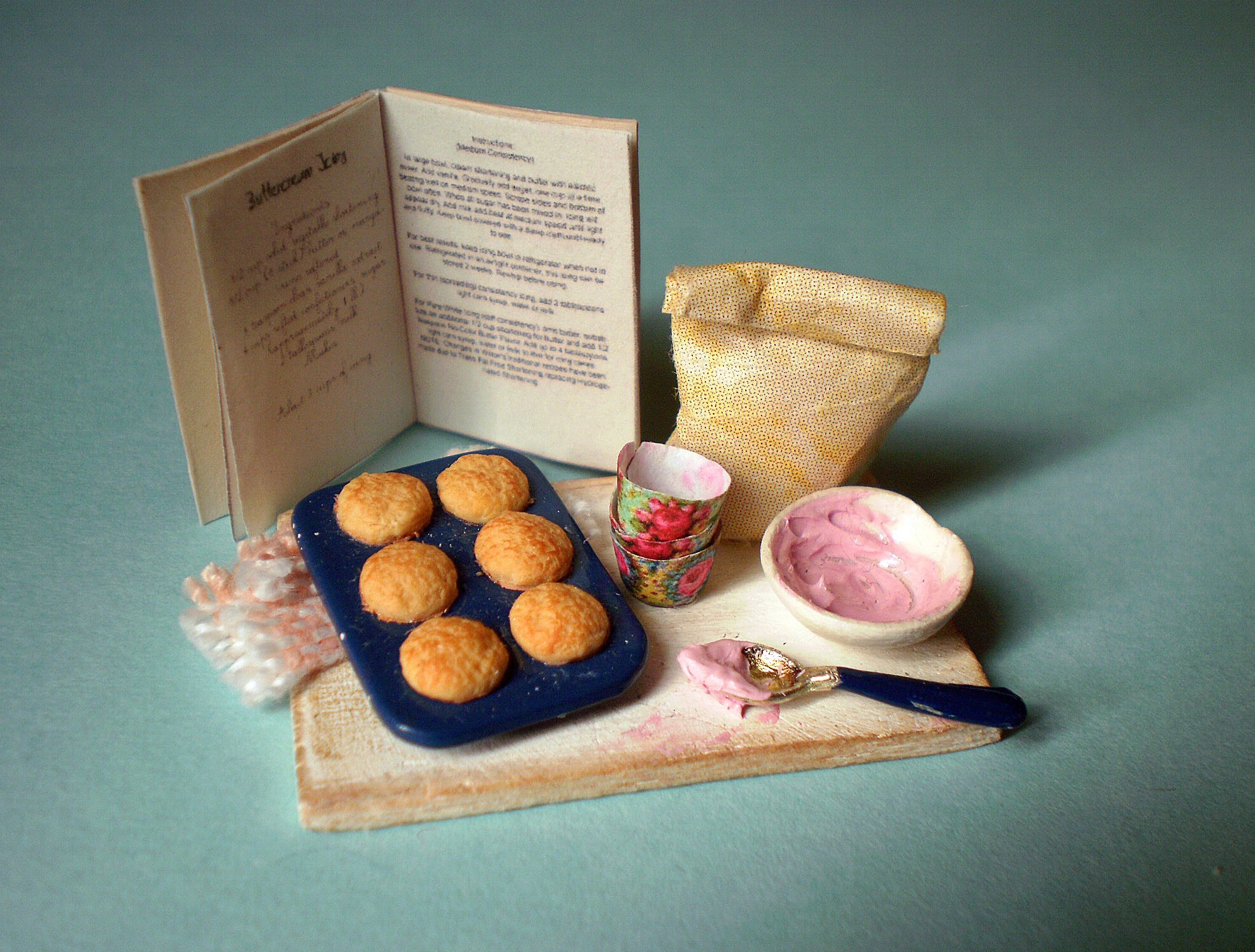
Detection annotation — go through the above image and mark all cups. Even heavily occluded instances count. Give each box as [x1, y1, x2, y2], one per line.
[607, 440, 731, 608]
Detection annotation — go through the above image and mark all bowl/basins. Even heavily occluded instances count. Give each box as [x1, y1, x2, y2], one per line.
[760, 485, 973, 648]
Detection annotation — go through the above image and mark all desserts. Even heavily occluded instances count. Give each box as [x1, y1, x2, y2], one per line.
[318, 422, 972, 756]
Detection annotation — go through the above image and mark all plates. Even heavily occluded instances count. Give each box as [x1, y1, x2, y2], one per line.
[291, 449, 649, 750]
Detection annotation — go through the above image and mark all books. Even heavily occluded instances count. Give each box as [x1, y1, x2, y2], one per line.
[131, 85, 644, 541]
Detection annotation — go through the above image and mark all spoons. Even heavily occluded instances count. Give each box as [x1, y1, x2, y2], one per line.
[687, 641, 1031, 730]
